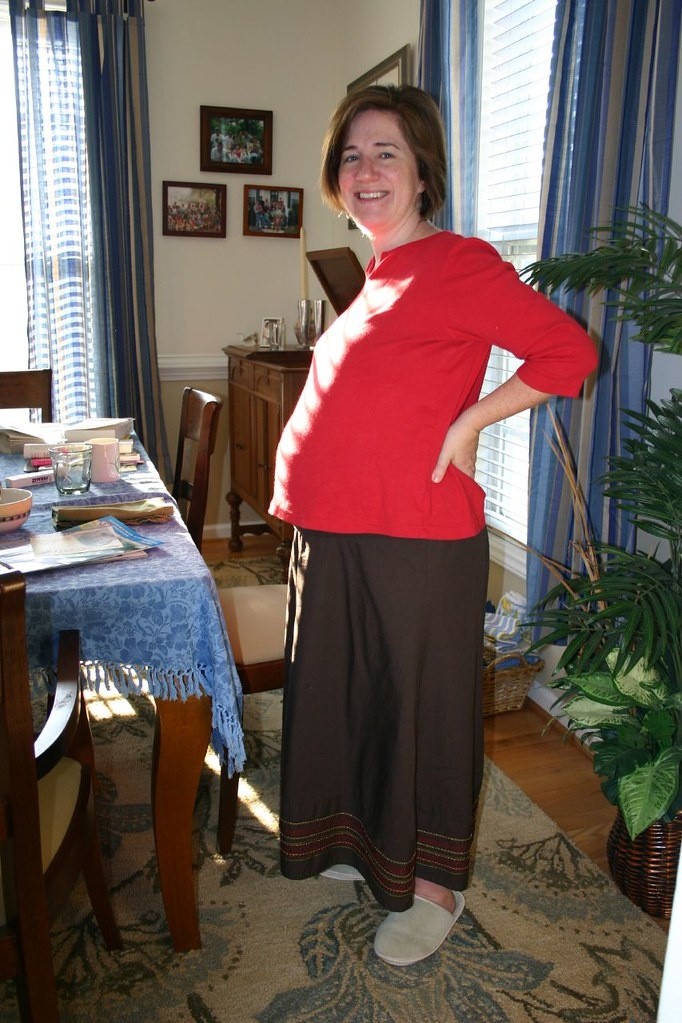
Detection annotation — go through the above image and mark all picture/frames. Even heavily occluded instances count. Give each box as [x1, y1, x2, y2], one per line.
[199, 105, 273, 175]
[260, 317, 283, 347]
[162, 180, 228, 238]
[347, 41, 411, 229]
[241, 184, 305, 238]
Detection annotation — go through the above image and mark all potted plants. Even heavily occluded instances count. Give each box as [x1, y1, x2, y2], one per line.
[557, 650, 682, 920]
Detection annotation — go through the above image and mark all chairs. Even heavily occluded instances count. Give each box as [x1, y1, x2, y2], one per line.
[0, 570, 124, 1023]
[0, 367, 52, 423]
[211, 584, 288, 856]
[173, 385, 223, 554]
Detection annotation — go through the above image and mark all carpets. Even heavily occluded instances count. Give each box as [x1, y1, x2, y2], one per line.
[0, 554, 668, 1023]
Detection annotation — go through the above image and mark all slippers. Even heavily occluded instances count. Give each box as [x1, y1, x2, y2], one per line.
[373, 889, 465, 966]
[319, 864, 366, 881]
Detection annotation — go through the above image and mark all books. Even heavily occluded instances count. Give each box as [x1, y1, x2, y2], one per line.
[0, 415, 145, 472]
[0, 515, 163, 575]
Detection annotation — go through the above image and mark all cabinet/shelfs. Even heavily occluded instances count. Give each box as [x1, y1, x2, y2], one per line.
[222, 345, 315, 584]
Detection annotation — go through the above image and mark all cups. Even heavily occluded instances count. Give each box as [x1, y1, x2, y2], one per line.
[85, 438, 121, 483]
[47, 445, 93, 495]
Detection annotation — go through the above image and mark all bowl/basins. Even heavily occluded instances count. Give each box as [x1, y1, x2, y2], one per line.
[0, 488, 32, 535]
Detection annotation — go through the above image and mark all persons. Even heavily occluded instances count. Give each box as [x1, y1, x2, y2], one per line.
[253, 195, 286, 232]
[210, 127, 263, 165]
[167, 200, 221, 232]
[266, 83, 599, 966]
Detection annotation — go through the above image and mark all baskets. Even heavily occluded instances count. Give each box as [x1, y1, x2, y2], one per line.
[481, 653, 546, 716]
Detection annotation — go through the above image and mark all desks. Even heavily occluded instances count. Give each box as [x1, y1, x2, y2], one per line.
[0, 423, 248, 953]
